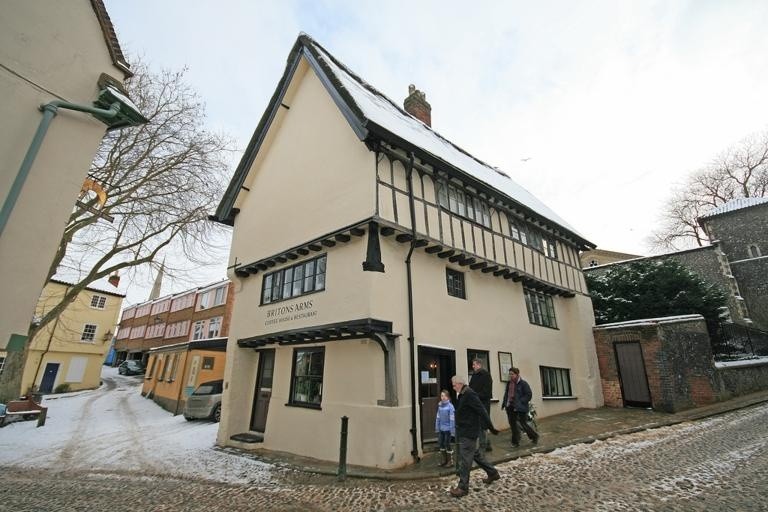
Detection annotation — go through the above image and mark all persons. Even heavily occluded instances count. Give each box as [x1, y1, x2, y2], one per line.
[450, 376, 501, 498]
[434, 390, 455, 467]
[501, 367, 539, 447]
[469, 358, 493, 452]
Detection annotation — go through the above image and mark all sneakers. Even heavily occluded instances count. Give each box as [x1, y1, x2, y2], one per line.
[510, 441, 520, 448]
[532, 433, 538, 444]
[483, 471, 501, 484]
[450, 487, 468, 497]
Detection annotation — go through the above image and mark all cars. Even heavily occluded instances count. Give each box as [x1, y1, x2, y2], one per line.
[183, 379, 223, 422]
[119, 359, 145, 375]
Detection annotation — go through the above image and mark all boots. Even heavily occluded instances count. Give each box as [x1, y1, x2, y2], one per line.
[443, 450, 454, 468]
[438, 449, 447, 467]
